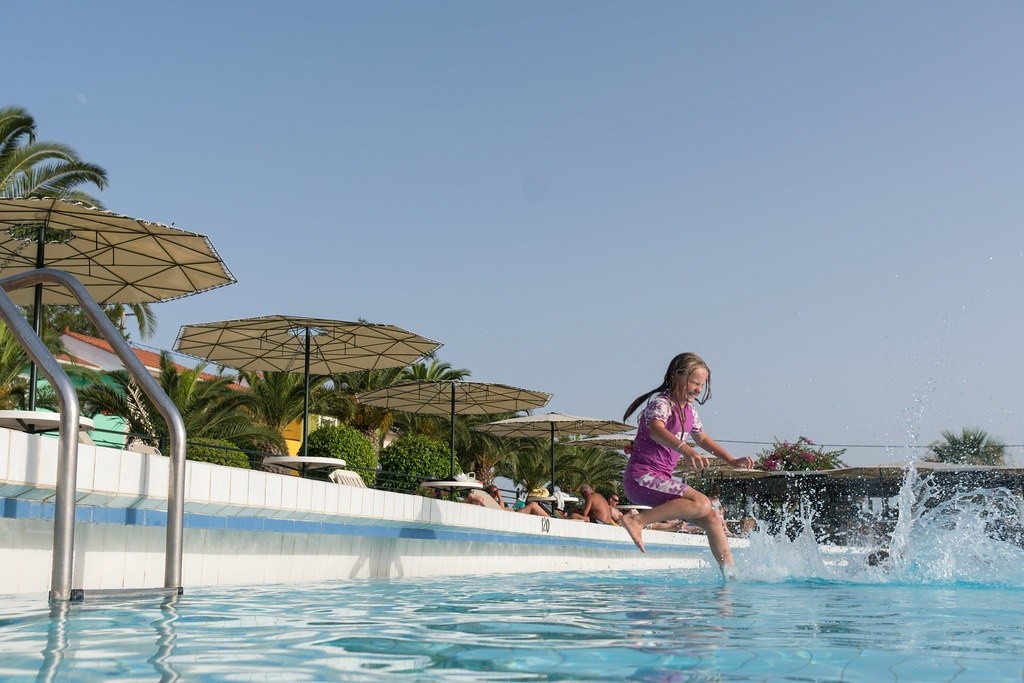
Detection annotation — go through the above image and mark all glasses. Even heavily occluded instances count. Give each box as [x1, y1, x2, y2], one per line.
[487, 490, 497, 495]
[610, 497, 619, 502]
[580, 491, 589, 496]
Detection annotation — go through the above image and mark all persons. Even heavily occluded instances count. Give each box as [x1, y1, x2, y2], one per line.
[619, 352, 755, 579]
[462, 492, 486, 505]
[571, 483, 611, 524]
[484, 484, 552, 518]
[605, 492, 682, 531]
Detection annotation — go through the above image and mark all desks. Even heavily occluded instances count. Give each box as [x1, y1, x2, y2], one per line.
[263, 456, 347, 478]
[420, 480, 484, 502]
[528, 496, 579, 518]
[0, 410, 96, 441]
[613, 503, 653, 517]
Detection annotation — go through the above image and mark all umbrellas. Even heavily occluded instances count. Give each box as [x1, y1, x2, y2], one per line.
[467, 409, 638, 519]
[355, 377, 556, 504]
[170, 311, 444, 473]
[1, 193, 238, 431]
[673, 445, 1024, 541]
